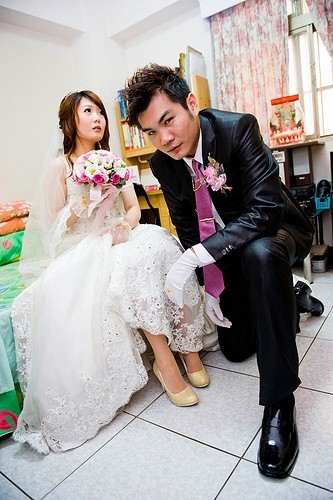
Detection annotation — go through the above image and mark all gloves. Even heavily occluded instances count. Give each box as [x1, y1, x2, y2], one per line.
[164, 247, 200, 310]
[202, 285, 232, 329]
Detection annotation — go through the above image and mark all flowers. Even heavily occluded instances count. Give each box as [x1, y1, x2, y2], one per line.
[72, 149, 132, 229]
[199, 156, 233, 195]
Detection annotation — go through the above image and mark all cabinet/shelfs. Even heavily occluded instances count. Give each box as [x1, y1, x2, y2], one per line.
[268, 140, 329, 284]
[114, 73, 212, 238]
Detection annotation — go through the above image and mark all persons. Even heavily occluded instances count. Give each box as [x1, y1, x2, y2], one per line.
[46, 88, 212, 408]
[122, 65, 325, 480]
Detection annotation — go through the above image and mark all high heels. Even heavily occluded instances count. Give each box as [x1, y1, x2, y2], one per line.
[178, 350, 210, 388]
[152, 359, 200, 407]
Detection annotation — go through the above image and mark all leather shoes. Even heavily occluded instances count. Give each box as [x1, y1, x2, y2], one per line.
[257, 395, 300, 479]
[293, 281, 324, 317]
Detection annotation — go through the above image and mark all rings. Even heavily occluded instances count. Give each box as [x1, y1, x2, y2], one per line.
[166, 289, 172, 293]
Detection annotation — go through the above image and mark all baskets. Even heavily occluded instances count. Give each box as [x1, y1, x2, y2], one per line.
[314, 189, 332, 210]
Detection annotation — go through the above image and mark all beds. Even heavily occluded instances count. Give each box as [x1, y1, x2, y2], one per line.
[1, 261, 51, 436]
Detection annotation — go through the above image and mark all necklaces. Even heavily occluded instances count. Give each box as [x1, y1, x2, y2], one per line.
[191, 170, 206, 192]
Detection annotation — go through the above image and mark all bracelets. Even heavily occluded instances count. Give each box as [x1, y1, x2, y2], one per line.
[81, 195, 89, 208]
[115, 223, 129, 231]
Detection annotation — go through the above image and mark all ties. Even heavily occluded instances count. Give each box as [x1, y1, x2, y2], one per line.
[191, 159, 225, 298]
